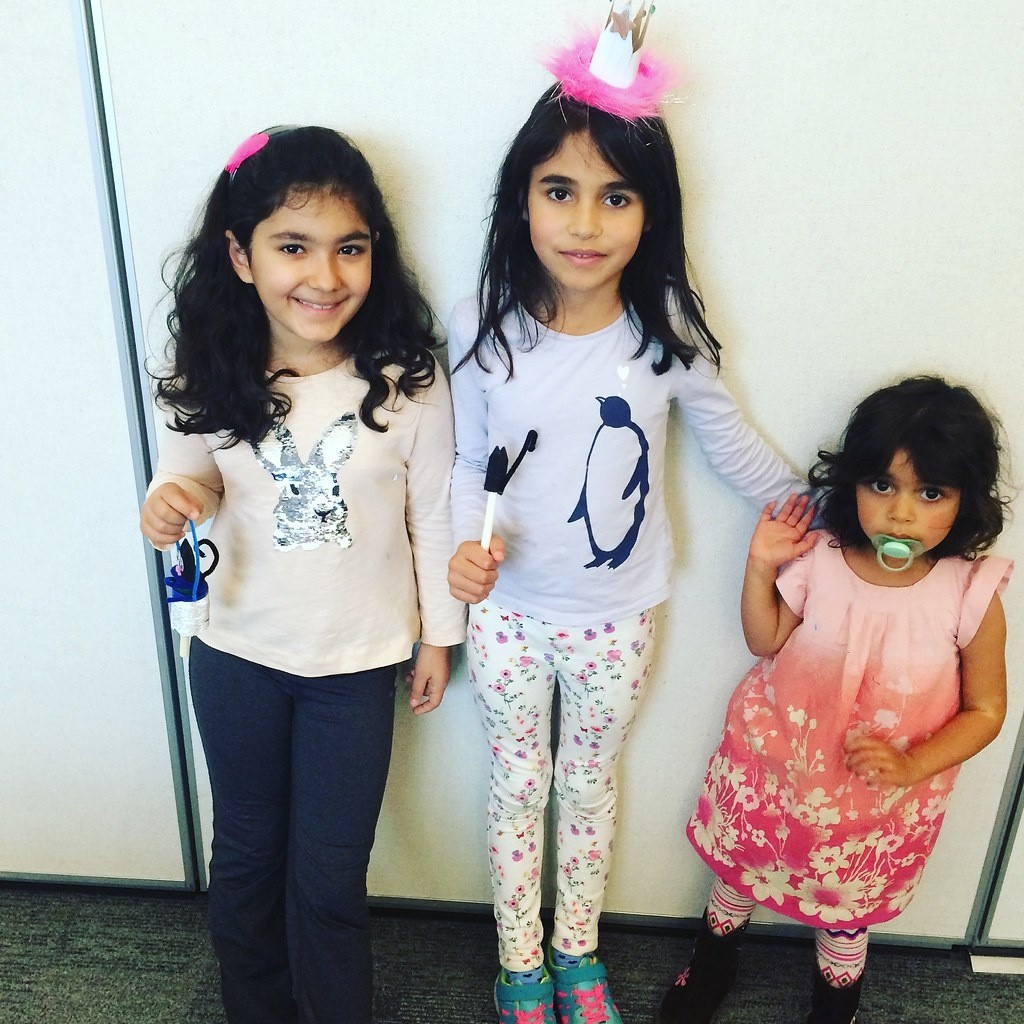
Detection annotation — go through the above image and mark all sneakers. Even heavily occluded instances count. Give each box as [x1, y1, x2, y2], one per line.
[547, 942, 622, 1024]
[494, 964, 557, 1024]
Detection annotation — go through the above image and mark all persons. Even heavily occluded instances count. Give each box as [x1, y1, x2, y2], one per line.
[446, 55, 833, 1024]
[655, 374, 1015, 1024]
[132, 121, 461, 1021]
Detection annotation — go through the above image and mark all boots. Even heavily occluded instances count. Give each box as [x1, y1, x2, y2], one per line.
[801, 960, 869, 1024]
[663, 911, 751, 1021]
